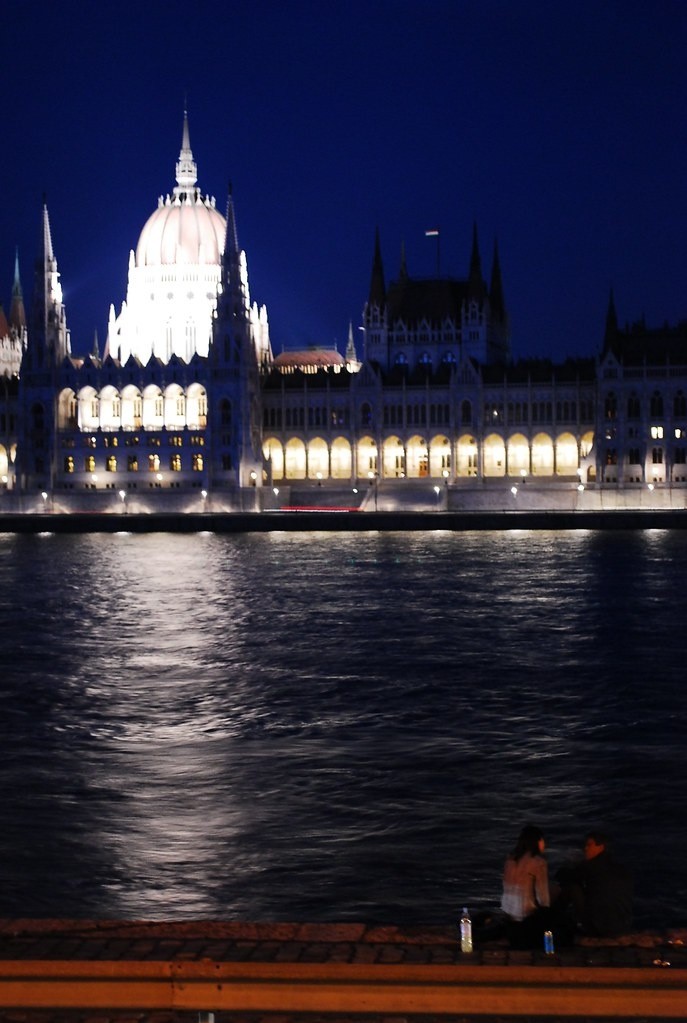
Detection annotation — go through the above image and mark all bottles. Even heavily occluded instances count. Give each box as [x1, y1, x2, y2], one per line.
[461, 908, 472, 952]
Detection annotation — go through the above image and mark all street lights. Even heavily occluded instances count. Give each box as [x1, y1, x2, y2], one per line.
[577, 468, 584, 482]
[443, 469, 450, 486]
[520, 469, 527, 483]
[368, 472, 379, 510]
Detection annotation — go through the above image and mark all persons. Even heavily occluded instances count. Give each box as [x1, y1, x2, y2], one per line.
[570, 832, 634, 938]
[501, 825, 572, 950]
